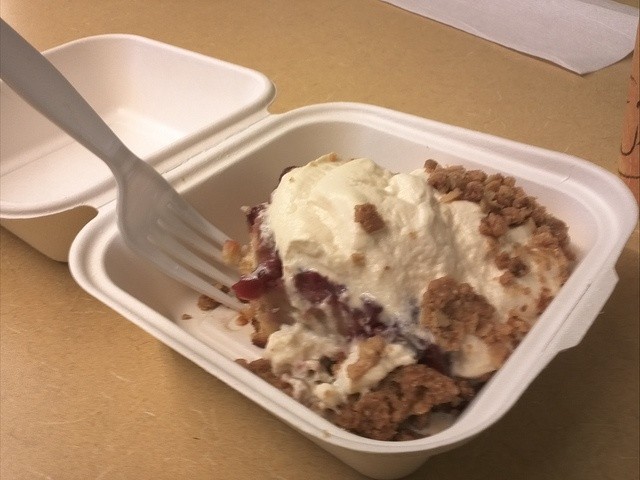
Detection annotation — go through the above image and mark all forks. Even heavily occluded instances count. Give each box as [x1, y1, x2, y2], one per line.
[0, 18, 251, 312]
